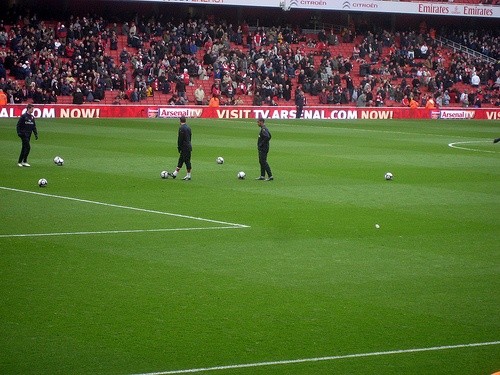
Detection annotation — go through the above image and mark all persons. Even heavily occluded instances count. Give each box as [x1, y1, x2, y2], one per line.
[168, 116, 192, 180]
[0, 6, 500, 119]
[16, 104, 38, 167]
[257, 118, 273, 181]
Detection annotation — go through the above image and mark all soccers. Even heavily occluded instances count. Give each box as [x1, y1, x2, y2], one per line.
[160, 171, 168, 178]
[238, 171, 246, 179]
[54, 156, 61, 163]
[57, 159, 64, 166]
[384, 172, 393, 181]
[216, 157, 224, 164]
[37, 178, 47, 188]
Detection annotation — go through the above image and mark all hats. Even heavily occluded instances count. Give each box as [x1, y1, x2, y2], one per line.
[25, 61, 29, 63]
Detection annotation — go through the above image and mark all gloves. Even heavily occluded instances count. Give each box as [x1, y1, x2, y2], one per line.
[18, 133, 22, 137]
[35, 134, 38, 139]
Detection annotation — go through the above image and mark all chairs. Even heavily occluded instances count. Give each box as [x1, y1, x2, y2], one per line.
[2, 22, 498, 107]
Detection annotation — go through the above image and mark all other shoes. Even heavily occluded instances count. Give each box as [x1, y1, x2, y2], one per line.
[182, 176, 191, 180]
[18, 163, 22, 167]
[266, 176, 273, 181]
[168, 173, 176, 178]
[256, 176, 265, 180]
[22, 162, 31, 167]
